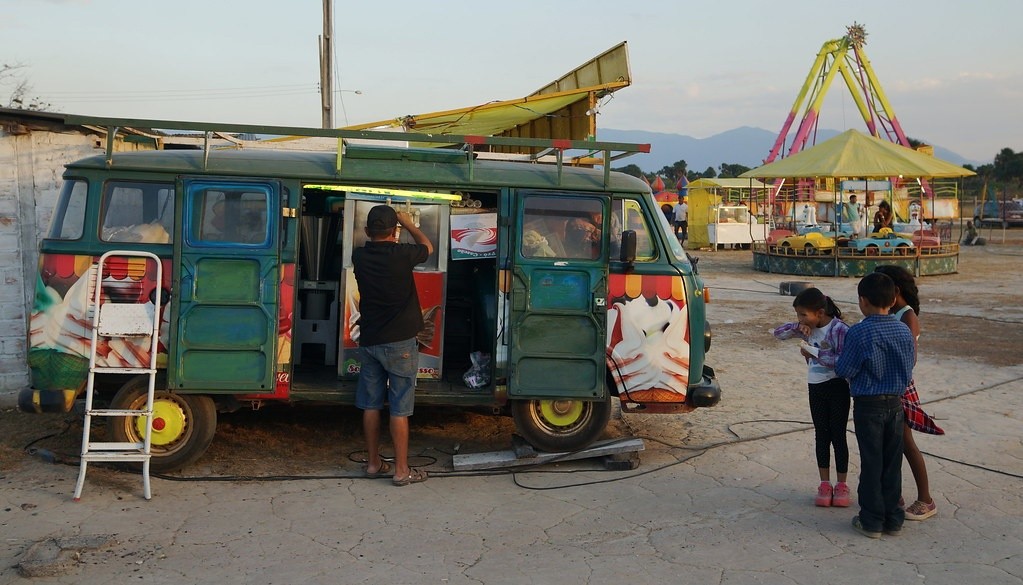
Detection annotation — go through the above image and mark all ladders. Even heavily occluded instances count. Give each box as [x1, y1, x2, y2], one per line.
[73, 249, 163, 503]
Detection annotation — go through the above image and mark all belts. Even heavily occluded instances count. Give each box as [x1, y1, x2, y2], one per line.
[853, 395, 900, 400]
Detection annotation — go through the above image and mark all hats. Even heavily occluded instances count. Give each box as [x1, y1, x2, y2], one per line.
[367, 206, 398, 229]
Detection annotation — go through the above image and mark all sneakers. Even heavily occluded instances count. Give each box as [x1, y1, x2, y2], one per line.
[832, 482, 848, 506]
[904, 498, 937, 520]
[852, 515, 881, 538]
[816, 482, 833, 507]
[882, 522, 902, 535]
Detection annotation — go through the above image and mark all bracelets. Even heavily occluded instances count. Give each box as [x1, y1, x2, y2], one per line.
[881, 217, 884, 220]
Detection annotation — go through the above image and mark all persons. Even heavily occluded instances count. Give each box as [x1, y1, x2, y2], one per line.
[592, 211, 622, 259]
[720, 195, 747, 249]
[670, 195, 688, 247]
[774, 288, 850, 507]
[876, 264, 945, 521]
[836, 274, 915, 537]
[843, 195, 862, 238]
[873, 201, 894, 233]
[964, 221, 977, 245]
[351, 205, 434, 486]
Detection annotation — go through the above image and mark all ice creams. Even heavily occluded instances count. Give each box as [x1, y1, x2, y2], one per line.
[349, 294, 361, 347]
[800, 340, 810, 364]
[415, 305, 442, 352]
[605, 294, 697, 405]
[27, 263, 173, 370]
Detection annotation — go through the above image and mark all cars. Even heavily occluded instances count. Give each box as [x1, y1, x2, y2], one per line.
[767, 227, 797, 251]
[973, 202, 1023, 229]
[912, 230, 943, 252]
[777, 231, 835, 255]
[799, 227, 848, 239]
[849, 234, 914, 256]
[867, 228, 913, 240]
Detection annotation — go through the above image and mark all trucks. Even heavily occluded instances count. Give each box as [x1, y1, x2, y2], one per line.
[15, 117, 721, 455]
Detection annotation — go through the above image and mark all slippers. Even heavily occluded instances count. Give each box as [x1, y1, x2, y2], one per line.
[392, 467, 427, 486]
[366, 461, 394, 478]
